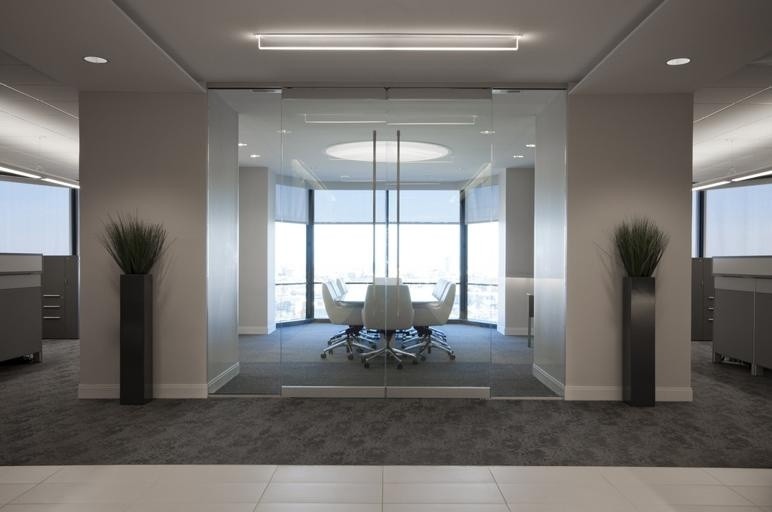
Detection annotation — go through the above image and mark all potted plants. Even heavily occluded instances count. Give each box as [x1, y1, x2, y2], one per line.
[610, 215, 671, 408]
[90, 205, 179, 406]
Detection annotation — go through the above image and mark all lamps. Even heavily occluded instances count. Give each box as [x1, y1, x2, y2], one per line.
[42, 177, 81, 190]
[692, 168, 772, 191]
[253, 31, 523, 51]
[0, 166, 42, 180]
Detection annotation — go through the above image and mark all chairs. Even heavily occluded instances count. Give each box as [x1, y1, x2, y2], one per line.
[320, 276, 456, 370]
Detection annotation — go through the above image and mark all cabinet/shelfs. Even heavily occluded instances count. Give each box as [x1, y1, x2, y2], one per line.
[711, 256, 772, 376]
[0, 253, 44, 364]
